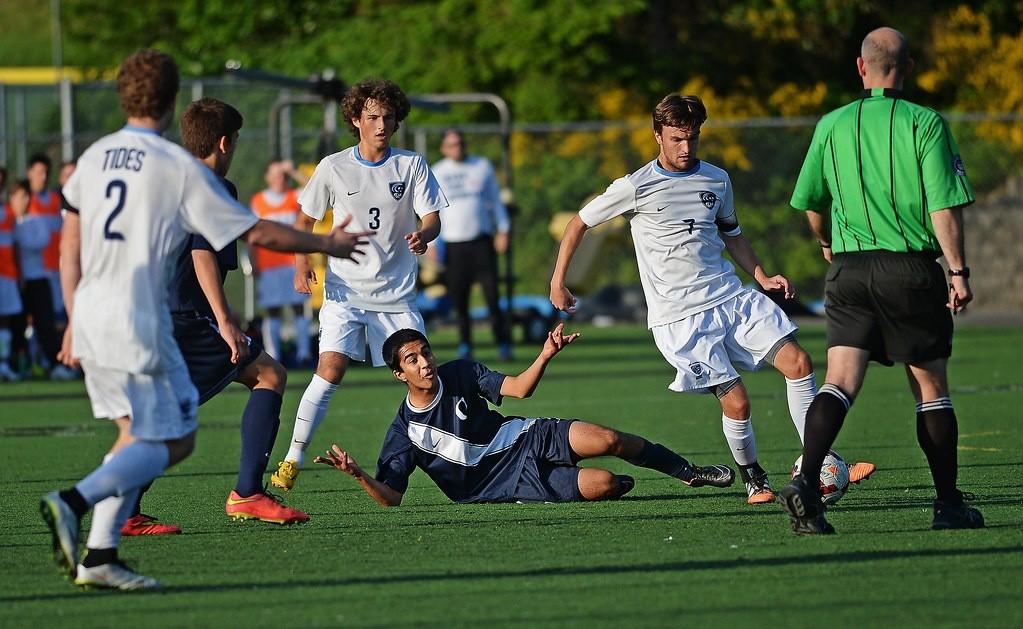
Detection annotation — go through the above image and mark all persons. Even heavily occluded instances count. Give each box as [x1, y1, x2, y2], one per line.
[430, 126, 514, 361]
[551, 93, 875, 504]
[312, 322, 734, 504]
[269, 78, 450, 492]
[121, 97, 309, 536]
[250, 159, 311, 368]
[37, 48, 377, 590]
[778, 28, 986, 534]
[0, 153, 81, 382]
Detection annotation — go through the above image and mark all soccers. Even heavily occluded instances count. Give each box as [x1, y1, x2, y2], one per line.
[790, 450, 850, 507]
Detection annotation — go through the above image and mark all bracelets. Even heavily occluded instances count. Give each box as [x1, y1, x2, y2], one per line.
[817, 237, 831, 248]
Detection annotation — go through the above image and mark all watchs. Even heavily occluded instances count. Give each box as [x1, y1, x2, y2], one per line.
[947, 267, 971, 278]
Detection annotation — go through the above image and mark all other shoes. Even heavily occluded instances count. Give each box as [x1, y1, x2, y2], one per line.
[458, 343, 473, 359]
[49, 366, 79, 381]
[499, 347, 512, 361]
[0, 364, 18, 382]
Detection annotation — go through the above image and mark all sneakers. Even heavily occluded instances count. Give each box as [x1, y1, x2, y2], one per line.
[743, 471, 775, 503]
[779, 479, 836, 536]
[36, 491, 79, 580]
[680, 462, 737, 487]
[845, 462, 877, 485]
[225, 489, 311, 526]
[931, 489, 985, 529]
[608, 474, 635, 499]
[120, 513, 182, 535]
[270, 460, 300, 491]
[74, 560, 163, 593]
